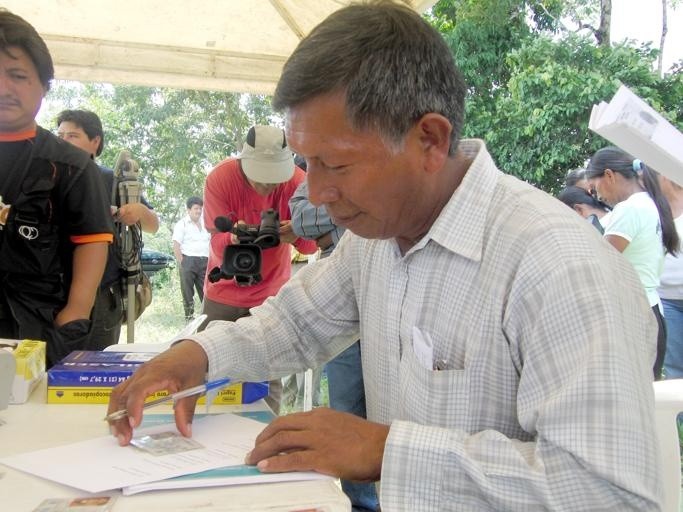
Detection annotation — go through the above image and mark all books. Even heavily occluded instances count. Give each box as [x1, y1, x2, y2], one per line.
[45, 350, 269, 405]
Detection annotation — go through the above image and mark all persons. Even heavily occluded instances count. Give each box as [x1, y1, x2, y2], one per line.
[171, 195, 212, 319]
[557, 145, 682, 380]
[0, 11, 114, 370]
[196, 124, 320, 414]
[56, 109, 159, 347]
[288, 178, 380, 512]
[107, 0, 683, 512]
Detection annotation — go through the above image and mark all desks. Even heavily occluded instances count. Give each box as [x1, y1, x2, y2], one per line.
[0, 371, 354, 511]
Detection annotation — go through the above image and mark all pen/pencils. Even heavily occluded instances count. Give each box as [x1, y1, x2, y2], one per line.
[104, 378, 230, 421]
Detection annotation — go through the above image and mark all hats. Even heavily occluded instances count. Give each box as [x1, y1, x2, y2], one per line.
[237, 124, 295, 186]
